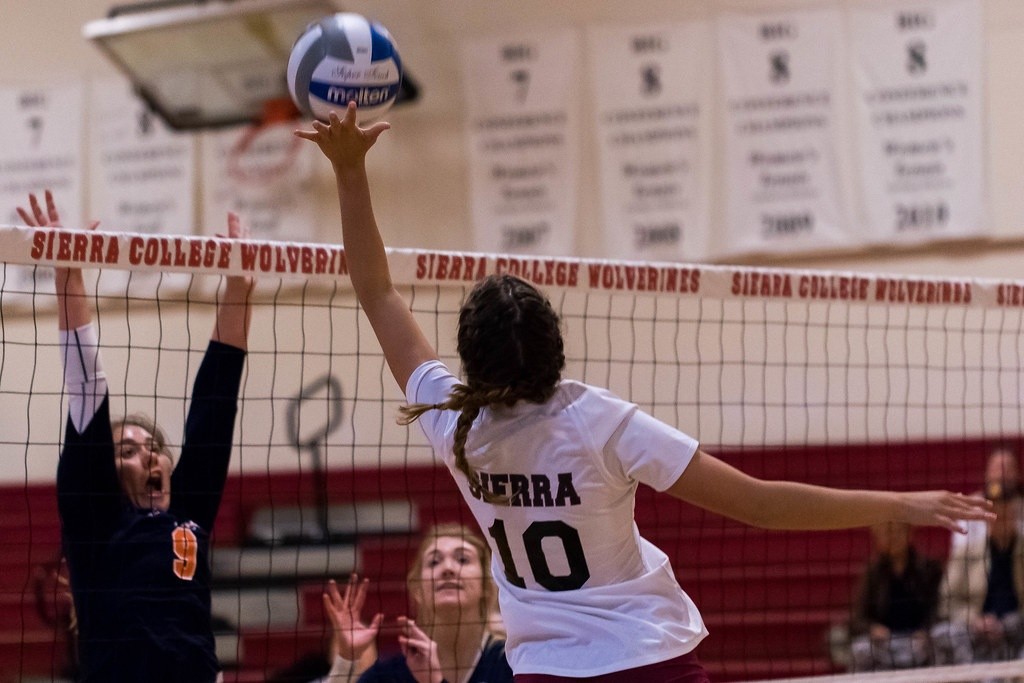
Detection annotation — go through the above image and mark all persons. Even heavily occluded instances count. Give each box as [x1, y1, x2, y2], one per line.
[293, 102, 998, 683]
[323, 524, 514, 683]
[16, 188, 256, 682]
[850, 449, 1024, 672]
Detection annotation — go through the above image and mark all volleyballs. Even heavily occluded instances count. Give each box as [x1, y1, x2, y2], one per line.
[284, 10, 404, 127]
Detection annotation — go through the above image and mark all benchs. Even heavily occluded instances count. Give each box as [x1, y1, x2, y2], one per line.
[0, 435, 1024, 682]
